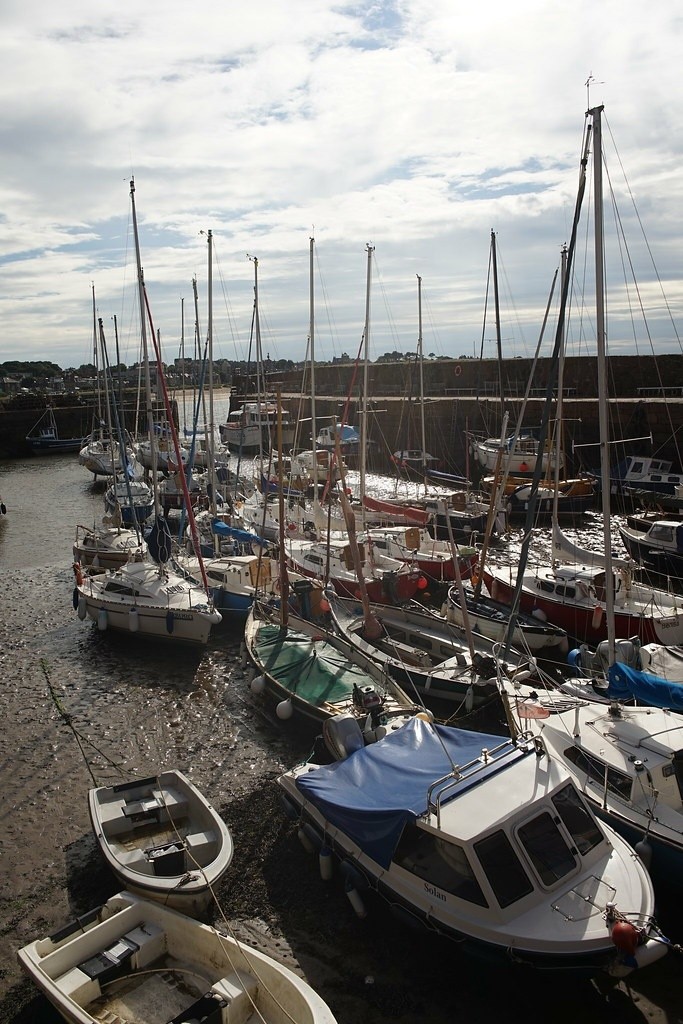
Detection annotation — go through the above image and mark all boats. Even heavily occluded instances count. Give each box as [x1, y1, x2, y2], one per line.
[268, 707, 656, 995]
[86, 769, 237, 913]
[16, 889, 338, 1024]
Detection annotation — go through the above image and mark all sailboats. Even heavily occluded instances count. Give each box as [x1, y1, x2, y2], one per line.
[22, 69, 683, 864]
